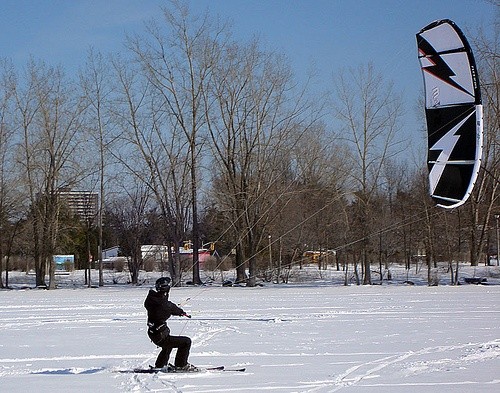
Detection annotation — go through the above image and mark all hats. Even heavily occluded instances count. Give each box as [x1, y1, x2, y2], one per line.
[155, 277, 171, 291]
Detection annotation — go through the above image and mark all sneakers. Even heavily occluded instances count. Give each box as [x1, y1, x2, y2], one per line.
[155, 366, 176, 373]
[175, 363, 199, 372]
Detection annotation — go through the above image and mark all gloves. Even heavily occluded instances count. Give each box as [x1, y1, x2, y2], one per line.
[178, 308, 183, 316]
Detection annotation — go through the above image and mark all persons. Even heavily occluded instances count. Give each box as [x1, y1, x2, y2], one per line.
[144, 277, 197, 372]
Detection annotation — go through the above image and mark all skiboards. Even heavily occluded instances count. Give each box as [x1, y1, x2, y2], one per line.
[120, 366, 246, 372]
